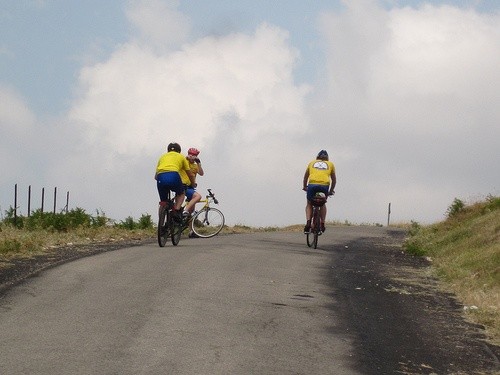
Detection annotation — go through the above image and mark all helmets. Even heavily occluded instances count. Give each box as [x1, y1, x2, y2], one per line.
[317, 149, 328, 159]
[167, 142, 181, 152]
[187, 148, 200, 155]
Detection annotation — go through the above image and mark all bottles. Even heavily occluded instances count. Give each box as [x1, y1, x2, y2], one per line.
[191, 210, 198, 218]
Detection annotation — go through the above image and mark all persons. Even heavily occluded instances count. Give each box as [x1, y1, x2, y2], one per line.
[154, 142, 197, 234]
[184, 147, 204, 238]
[302, 149, 336, 233]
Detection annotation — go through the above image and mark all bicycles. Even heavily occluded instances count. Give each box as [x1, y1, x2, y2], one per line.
[172, 188, 225, 238]
[157, 182, 197, 247]
[302, 186, 334, 249]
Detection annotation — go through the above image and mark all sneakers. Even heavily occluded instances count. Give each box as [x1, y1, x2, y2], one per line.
[321, 224, 325, 233]
[304, 225, 311, 234]
[183, 207, 189, 217]
[170, 209, 184, 223]
[188, 230, 201, 238]
